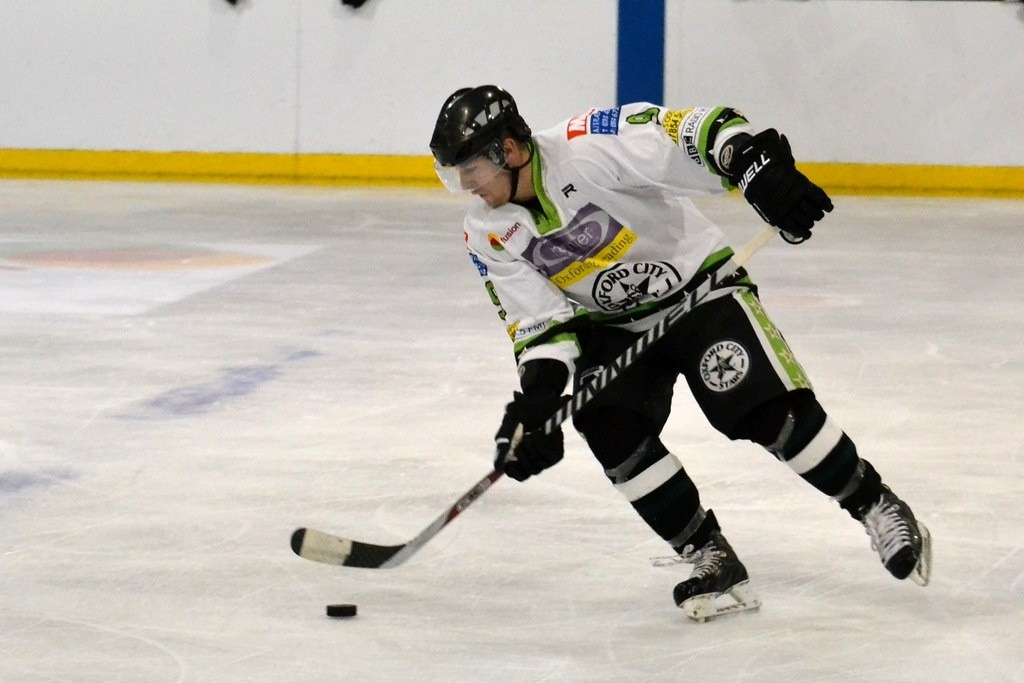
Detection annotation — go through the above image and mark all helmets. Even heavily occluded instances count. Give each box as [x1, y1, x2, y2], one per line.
[428, 85, 531, 168]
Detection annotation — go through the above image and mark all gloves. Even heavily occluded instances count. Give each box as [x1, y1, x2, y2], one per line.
[728, 128, 834, 245]
[496, 358, 569, 482]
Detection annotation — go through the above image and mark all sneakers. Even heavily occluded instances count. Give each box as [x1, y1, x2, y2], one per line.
[860, 479, 931, 587]
[653, 524, 763, 623]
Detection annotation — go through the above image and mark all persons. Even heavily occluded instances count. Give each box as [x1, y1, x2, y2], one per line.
[431, 84, 923, 614]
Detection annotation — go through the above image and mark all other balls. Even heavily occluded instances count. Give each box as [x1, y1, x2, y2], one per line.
[326, 604, 357, 617]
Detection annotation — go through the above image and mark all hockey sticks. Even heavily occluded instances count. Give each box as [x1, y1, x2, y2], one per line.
[291, 226, 781, 571]
